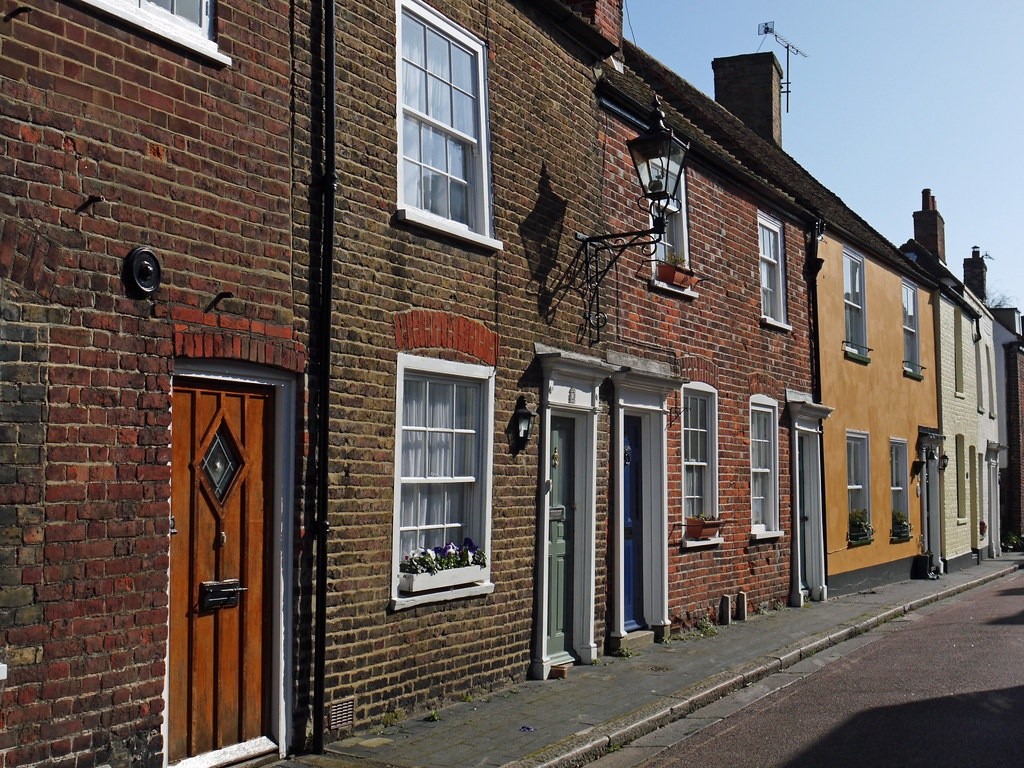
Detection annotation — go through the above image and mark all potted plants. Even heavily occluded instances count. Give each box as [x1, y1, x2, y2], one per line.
[891, 510, 909, 533]
[850, 508, 870, 539]
[657, 254, 685, 283]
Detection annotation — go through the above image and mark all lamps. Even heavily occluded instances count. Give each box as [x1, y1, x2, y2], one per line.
[940, 451, 949, 470]
[576, 93, 690, 342]
[504, 394, 537, 459]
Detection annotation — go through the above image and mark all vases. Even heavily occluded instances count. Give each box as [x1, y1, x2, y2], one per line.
[685, 515, 724, 540]
[398, 563, 487, 593]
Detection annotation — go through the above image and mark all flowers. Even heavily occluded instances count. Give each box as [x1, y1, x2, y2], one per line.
[397, 536, 487, 577]
